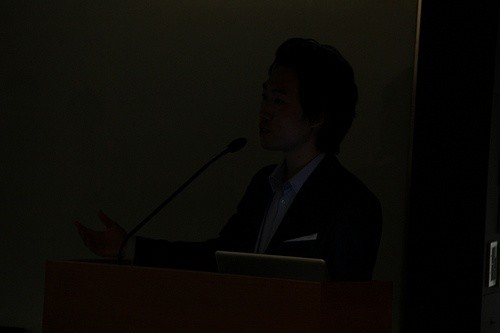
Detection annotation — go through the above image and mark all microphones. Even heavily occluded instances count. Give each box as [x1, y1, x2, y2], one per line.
[115, 139, 250, 265]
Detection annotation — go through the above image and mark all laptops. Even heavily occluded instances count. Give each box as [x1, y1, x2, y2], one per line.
[213, 250, 329, 281]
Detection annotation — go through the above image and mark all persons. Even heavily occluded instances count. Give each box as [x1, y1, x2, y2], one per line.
[75, 38, 386, 280]
